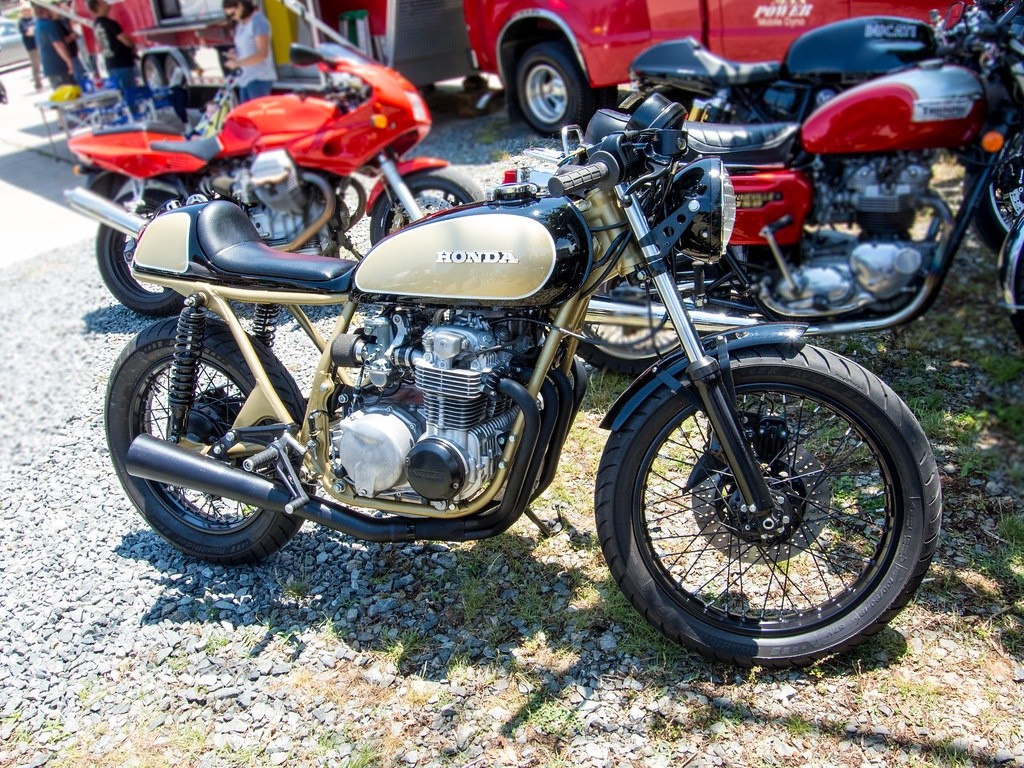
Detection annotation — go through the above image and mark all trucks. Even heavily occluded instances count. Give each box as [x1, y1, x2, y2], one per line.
[68, 0, 469, 120]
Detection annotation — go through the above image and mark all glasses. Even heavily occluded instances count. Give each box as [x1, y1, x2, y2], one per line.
[227, 13, 235, 17]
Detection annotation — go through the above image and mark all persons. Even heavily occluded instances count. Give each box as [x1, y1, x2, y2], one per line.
[18, 2, 42, 89]
[223, 0, 279, 105]
[87, 0, 140, 113]
[35, 1, 86, 129]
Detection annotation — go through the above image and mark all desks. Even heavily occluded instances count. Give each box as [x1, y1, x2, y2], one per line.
[35, 89, 133, 160]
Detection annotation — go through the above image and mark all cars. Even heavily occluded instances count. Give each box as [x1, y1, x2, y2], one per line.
[452, 0, 1024, 142]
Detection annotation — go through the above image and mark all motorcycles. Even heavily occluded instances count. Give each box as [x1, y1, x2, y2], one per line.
[59, 0, 1024, 378]
[98, 93, 944, 673]
